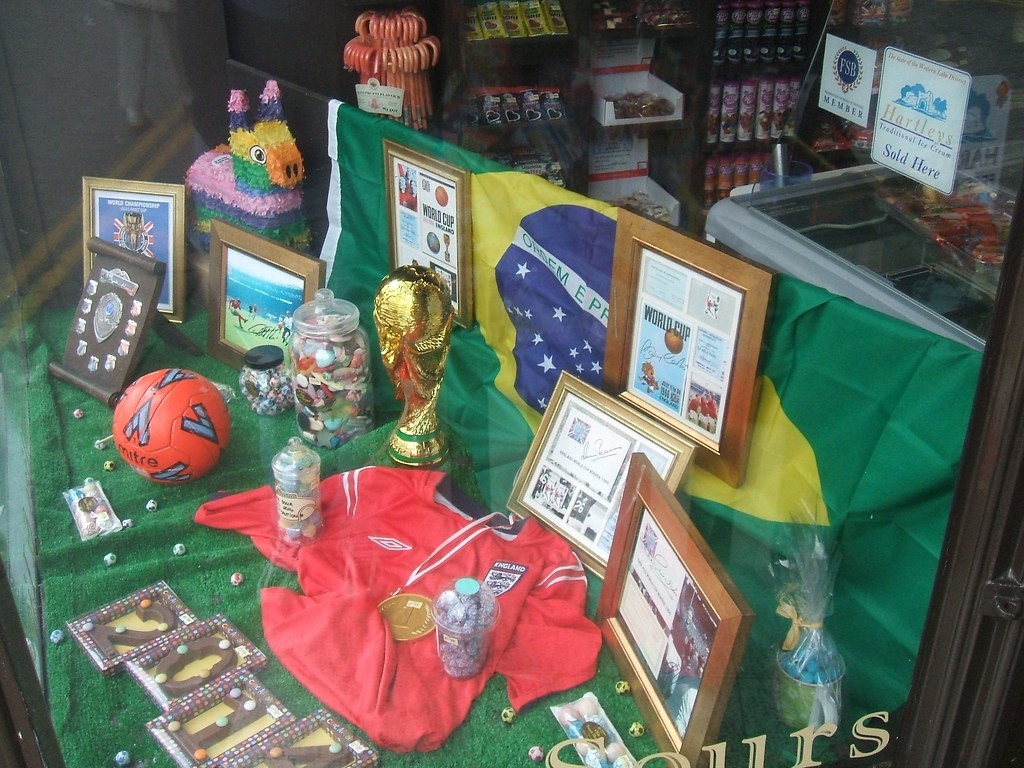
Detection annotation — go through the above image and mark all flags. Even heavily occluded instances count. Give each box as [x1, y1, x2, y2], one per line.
[319, 99, 984, 746]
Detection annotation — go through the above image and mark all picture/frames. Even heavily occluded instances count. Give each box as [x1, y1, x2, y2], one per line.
[506, 368, 702, 580]
[592, 452, 757, 768]
[601, 203, 777, 489]
[207, 218, 326, 374]
[381, 135, 474, 332]
[81, 176, 187, 325]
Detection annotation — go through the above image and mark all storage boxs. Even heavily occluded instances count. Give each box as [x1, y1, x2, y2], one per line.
[591, 70, 685, 126]
[587, 176, 680, 227]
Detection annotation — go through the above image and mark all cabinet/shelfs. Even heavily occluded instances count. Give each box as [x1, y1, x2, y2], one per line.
[416, 0, 699, 239]
[650, 0, 829, 232]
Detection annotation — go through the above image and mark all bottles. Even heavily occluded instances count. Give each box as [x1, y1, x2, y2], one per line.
[290, 288, 376, 449]
[271, 436, 325, 544]
[239, 345, 294, 416]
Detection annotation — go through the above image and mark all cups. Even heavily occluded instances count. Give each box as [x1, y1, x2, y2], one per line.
[428, 576, 501, 679]
[777, 649, 846, 732]
[760, 160, 813, 193]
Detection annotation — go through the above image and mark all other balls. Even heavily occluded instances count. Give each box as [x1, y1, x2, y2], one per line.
[113, 370, 229, 484]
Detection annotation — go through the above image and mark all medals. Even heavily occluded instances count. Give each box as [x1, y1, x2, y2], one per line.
[377, 593, 436, 644]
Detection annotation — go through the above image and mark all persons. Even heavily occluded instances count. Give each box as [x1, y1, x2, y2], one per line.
[282, 310, 293, 346]
[248, 303, 257, 320]
[688, 390, 717, 432]
[228, 299, 249, 329]
[678, 606, 694, 653]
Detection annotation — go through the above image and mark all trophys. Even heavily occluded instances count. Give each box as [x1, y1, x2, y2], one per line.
[372, 265, 455, 468]
